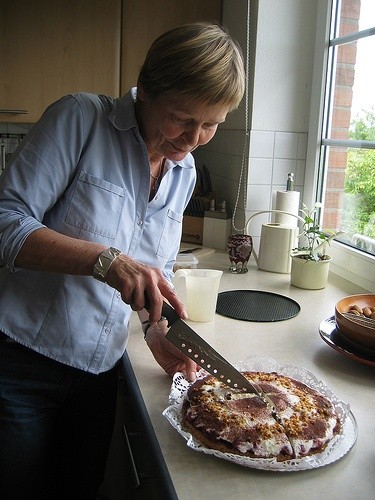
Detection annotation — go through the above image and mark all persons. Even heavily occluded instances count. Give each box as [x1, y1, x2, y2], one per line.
[0, 24, 244, 500]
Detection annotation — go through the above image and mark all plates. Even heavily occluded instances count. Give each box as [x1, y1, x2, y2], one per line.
[316, 410, 359, 470]
[318, 315, 375, 367]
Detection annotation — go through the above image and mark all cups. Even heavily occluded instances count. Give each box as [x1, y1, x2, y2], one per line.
[227, 233, 252, 275]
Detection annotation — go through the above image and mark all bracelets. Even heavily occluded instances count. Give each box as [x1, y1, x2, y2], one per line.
[142, 315, 168, 341]
[93, 246, 123, 283]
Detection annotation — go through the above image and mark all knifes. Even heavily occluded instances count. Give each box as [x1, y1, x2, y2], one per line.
[142, 294, 261, 397]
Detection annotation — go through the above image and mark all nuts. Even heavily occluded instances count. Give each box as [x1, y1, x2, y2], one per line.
[348, 305, 375, 321]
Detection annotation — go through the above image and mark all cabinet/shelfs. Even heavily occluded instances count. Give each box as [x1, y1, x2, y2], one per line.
[101, 355, 178, 500]
[1, 1, 222, 124]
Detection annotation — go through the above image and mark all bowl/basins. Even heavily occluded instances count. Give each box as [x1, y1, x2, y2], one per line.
[334, 294, 375, 352]
[173, 253, 199, 272]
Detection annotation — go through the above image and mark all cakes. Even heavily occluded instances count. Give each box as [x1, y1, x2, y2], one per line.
[181, 371, 342, 461]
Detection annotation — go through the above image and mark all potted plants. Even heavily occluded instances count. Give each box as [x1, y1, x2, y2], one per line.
[290, 200, 343, 289]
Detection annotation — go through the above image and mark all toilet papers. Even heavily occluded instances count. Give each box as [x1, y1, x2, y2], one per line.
[275, 191, 299, 248]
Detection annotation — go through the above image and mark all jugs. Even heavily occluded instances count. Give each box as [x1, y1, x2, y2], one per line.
[172, 268, 224, 322]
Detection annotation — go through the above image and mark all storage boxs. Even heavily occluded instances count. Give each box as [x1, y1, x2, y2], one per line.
[173, 253, 198, 273]
